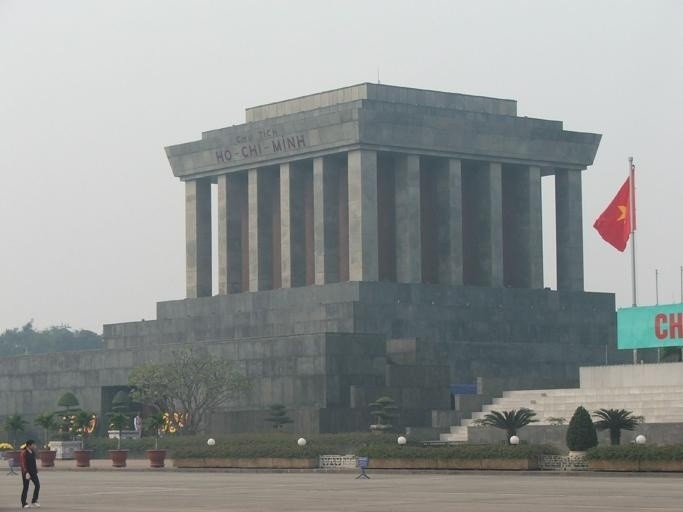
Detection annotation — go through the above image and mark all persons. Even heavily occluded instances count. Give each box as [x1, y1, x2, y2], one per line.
[134, 411, 142, 437]
[21, 439, 41, 507]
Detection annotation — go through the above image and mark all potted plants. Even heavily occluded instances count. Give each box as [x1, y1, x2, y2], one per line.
[34, 409, 65, 467]
[367, 395, 398, 436]
[104, 411, 131, 468]
[139, 411, 166, 468]
[4, 411, 29, 466]
[72, 409, 94, 467]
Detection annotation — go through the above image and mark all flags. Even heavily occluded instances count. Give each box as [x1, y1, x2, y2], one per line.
[592, 169, 636, 251]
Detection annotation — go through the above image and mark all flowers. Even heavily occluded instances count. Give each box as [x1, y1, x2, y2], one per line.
[0, 441, 13, 450]
